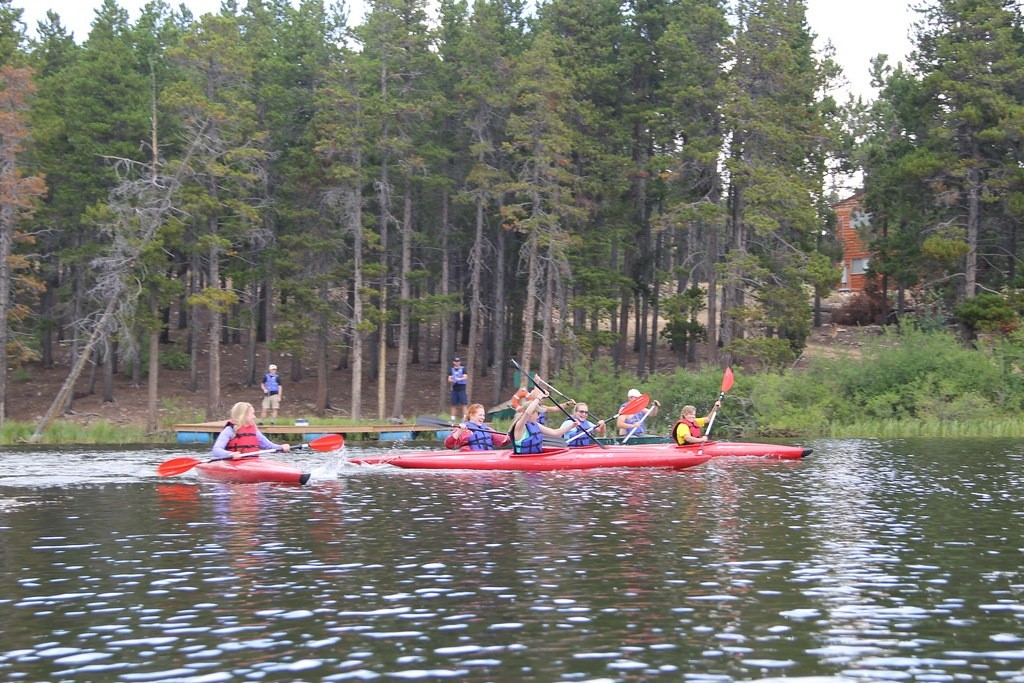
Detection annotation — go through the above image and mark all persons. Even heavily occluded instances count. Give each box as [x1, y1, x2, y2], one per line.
[445, 404, 511, 452]
[447, 358, 468, 424]
[258, 364, 283, 426]
[672, 401, 721, 447]
[562, 403, 606, 448]
[510, 389, 580, 455]
[530, 375, 576, 426]
[212, 402, 291, 460]
[617, 389, 661, 436]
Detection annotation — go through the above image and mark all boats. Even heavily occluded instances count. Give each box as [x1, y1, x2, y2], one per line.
[195, 460, 311, 486]
[346, 441, 813, 471]
[487, 396, 517, 419]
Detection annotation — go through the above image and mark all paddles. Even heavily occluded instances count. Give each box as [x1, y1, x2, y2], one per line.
[621, 402, 658, 444]
[157, 433, 345, 477]
[415, 414, 567, 449]
[567, 393, 650, 445]
[510, 357, 607, 449]
[697, 365, 735, 455]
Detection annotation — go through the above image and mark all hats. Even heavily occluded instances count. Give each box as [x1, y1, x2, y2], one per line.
[269, 364, 277, 370]
[628, 389, 642, 398]
[524, 401, 547, 413]
[452, 357, 461, 361]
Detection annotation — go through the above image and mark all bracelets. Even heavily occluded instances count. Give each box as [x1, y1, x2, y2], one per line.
[536, 397, 541, 401]
[566, 402, 569, 406]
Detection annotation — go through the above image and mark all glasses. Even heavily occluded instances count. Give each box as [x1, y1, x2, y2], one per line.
[577, 410, 589, 414]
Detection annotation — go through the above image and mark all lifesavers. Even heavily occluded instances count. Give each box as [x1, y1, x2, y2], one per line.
[512, 388, 530, 413]
